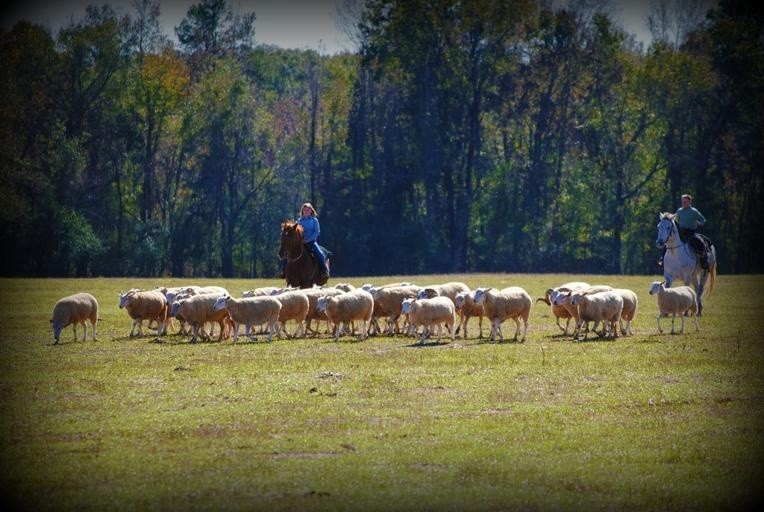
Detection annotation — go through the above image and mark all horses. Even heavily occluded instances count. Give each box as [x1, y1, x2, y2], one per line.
[654, 211, 718, 315]
[277, 220, 330, 286]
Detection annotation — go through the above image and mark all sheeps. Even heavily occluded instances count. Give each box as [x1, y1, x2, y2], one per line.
[648, 280, 700, 334]
[117, 280, 532, 343]
[535, 282, 638, 341]
[49, 292, 100, 343]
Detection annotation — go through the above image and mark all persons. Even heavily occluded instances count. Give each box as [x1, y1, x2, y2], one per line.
[674, 193, 708, 271]
[296, 202, 330, 280]
